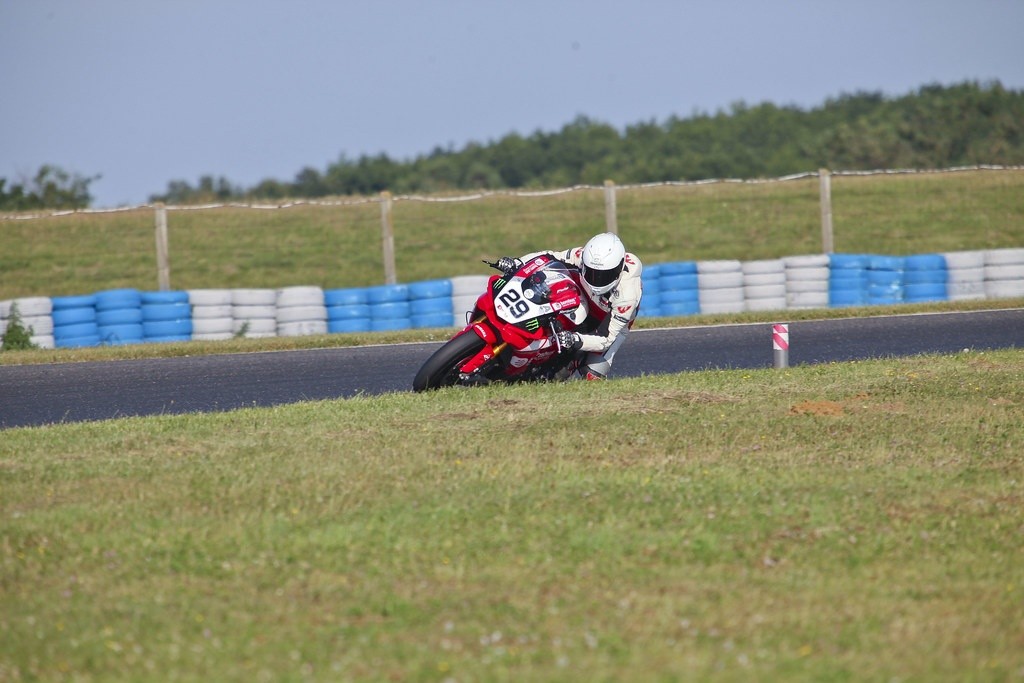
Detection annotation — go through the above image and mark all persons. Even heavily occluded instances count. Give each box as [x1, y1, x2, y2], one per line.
[498, 232, 643, 381]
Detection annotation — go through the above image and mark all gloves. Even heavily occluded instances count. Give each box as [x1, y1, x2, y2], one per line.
[496, 257, 525, 275]
[552, 330, 583, 355]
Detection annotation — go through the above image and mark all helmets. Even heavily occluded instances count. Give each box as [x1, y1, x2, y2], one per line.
[579, 232, 626, 296]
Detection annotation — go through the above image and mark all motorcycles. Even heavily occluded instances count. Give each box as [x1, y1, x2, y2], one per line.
[413, 254, 601, 393]
